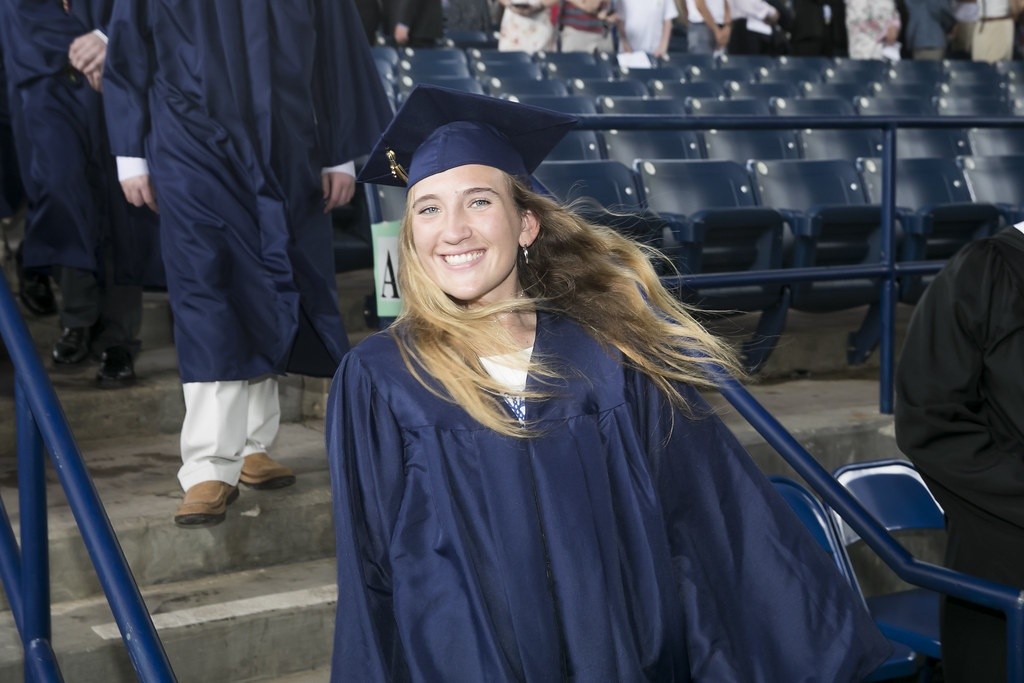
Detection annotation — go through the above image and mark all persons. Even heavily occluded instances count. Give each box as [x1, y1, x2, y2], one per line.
[325, 87, 891, 683]
[495, 0, 1024, 66]
[0, 0, 443, 524]
[896, 222, 1024, 683]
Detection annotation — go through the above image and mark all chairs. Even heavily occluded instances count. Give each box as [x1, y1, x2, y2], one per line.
[364, 49, 1024, 373]
[766, 459, 943, 683]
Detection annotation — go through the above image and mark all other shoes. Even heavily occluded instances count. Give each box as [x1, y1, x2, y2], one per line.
[240, 453, 296, 490]
[174, 481, 239, 524]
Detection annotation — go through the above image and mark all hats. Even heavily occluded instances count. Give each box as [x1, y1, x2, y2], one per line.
[354, 82, 579, 197]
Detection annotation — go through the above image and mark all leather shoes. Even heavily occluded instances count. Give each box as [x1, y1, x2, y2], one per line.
[16, 239, 58, 318]
[96, 346, 134, 388]
[51, 326, 91, 366]
